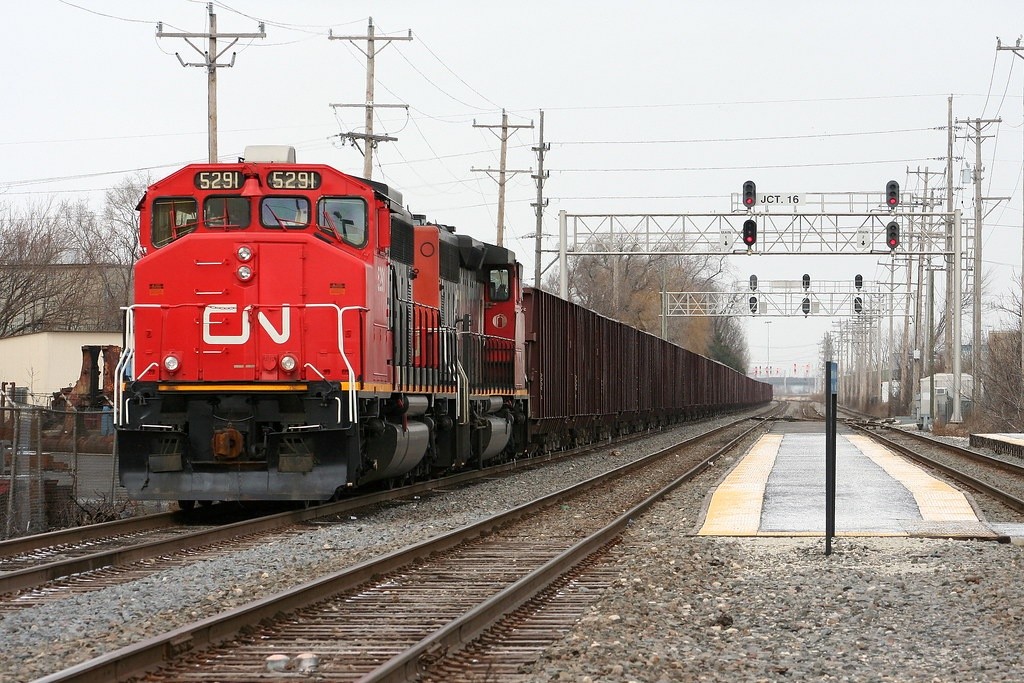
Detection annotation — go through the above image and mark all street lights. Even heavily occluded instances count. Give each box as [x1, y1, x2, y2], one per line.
[764, 321, 772, 384]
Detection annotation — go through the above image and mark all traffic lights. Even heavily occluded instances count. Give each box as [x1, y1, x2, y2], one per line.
[743, 219, 756, 245]
[743, 180, 756, 208]
[886, 222, 899, 248]
[886, 180, 899, 207]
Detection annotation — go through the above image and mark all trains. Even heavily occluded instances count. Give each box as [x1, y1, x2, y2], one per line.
[118, 146, 774, 512]
